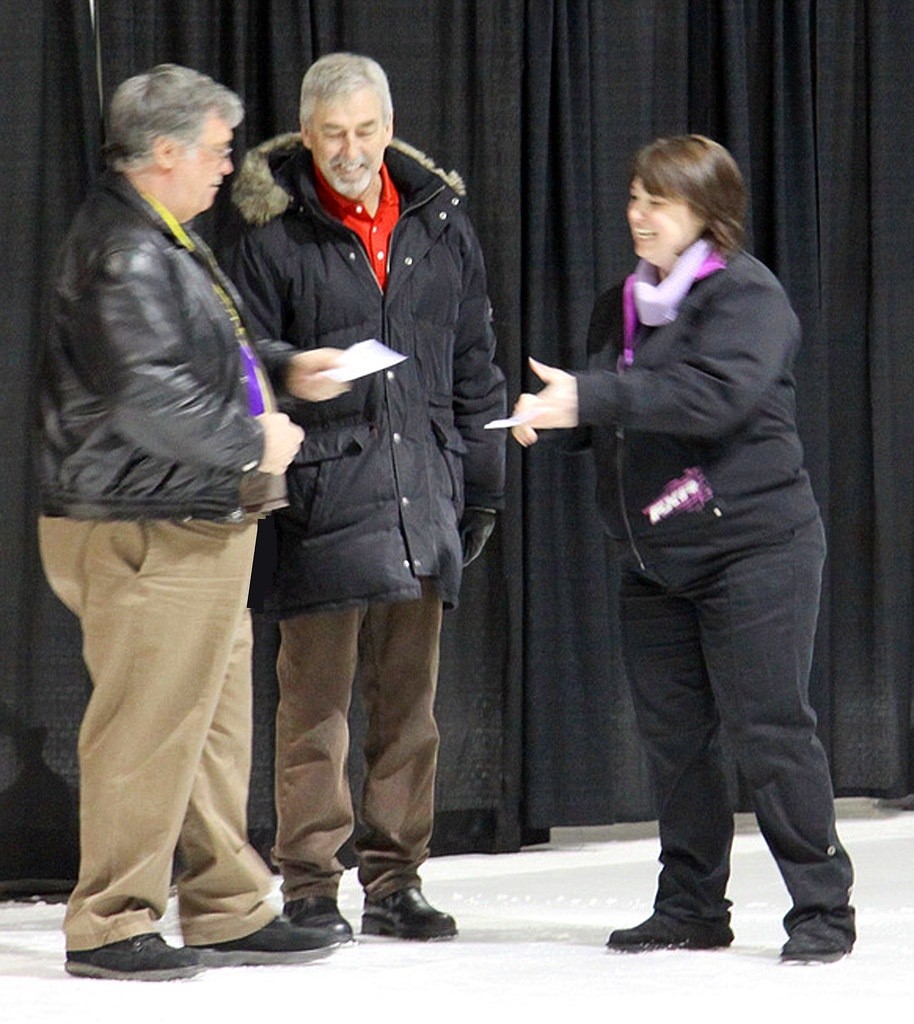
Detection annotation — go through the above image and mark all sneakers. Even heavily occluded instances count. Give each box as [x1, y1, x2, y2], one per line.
[191, 915, 337, 971]
[65, 928, 201, 982]
[605, 907, 738, 952]
[782, 909, 852, 964]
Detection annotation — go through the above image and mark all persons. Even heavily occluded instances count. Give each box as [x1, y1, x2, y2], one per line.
[509, 133, 858, 963]
[36, 64, 342, 981]
[218, 53, 510, 943]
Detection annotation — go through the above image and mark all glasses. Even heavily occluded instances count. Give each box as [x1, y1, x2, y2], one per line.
[182, 133, 233, 158]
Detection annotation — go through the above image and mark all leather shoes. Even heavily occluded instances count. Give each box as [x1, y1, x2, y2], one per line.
[287, 895, 354, 948]
[361, 884, 459, 940]
